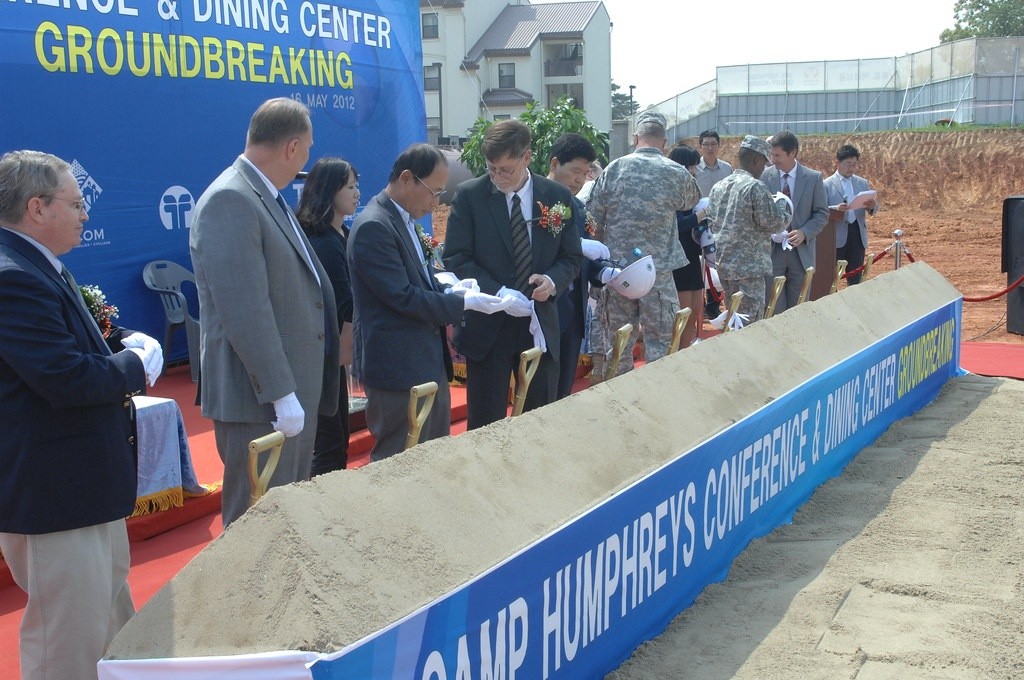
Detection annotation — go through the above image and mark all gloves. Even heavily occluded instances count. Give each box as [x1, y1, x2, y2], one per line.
[771, 231, 794, 251]
[121, 332, 164, 387]
[444, 278, 481, 296]
[581, 237, 611, 260]
[710, 309, 750, 331]
[602, 267, 622, 284]
[771, 190, 786, 204]
[270, 392, 305, 437]
[528, 301, 547, 352]
[495, 286, 533, 318]
[463, 292, 516, 314]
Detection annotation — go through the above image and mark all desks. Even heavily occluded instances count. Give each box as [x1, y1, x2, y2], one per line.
[130, 396, 218, 517]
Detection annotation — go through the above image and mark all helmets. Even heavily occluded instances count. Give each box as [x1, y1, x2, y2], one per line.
[691, 217, 714, 245]
[692, 197, 710, 214]
[700, 227, 717, 269]
[604, 255, 657, 300]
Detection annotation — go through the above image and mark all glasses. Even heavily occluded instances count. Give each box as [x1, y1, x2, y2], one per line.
[40, 193, 85, 214]
[701, 142, 719, 149]
[415, 175, 447, 199]
[482, 155, 522, 178]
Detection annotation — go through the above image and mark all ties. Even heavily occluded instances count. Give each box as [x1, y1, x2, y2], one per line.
[277, 193, 293, 230]
[509, 195, 533, 298]
[844, 177, 856, 224]
[58, 261, 115, 358]
[781, 174, 792, 233]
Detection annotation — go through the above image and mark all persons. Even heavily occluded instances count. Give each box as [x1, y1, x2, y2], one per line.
[190, 97, 341, 532]
[297, 108, 878, 474]
[0, 150, 166, 680]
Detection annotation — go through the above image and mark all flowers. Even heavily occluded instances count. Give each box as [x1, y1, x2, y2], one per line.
[80, 283, 121, 338]
[417, 224, 442, 269]
[520, 201, 572, 236]
[583, 207, 597, 237]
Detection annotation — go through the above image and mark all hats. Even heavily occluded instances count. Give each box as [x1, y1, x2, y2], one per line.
[740, 135, 774, 166]
[637, 109, 667, 133]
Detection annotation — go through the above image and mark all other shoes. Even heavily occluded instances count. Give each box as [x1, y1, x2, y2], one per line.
[183, 484, 209, 497]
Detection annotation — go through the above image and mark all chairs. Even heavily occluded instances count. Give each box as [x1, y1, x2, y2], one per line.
[144, 260, 201, 382]
